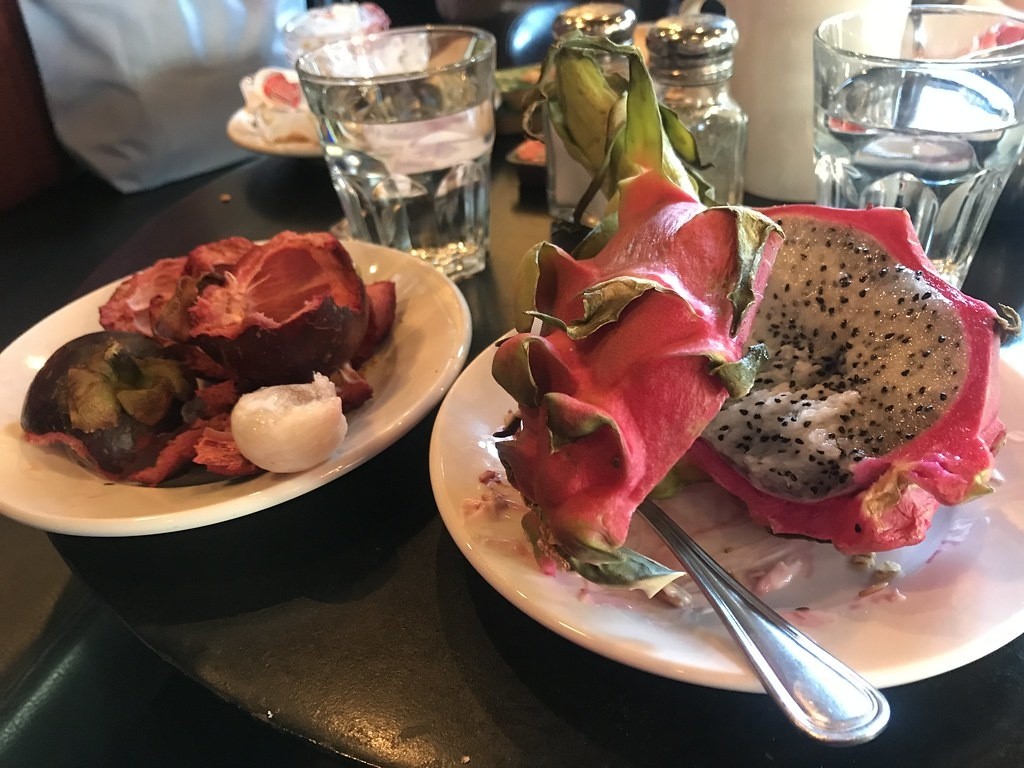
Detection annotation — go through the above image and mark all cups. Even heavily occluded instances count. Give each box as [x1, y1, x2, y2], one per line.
[281, 4, 390, 68]
[293, 23, 497, 282]
[808, 4, 1024, 297]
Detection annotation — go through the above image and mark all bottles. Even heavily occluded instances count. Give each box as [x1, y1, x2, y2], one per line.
[540, 3, 638, 259]
[630, 13, 751, 216]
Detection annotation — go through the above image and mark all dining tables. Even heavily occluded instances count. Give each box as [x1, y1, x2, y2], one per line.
[46, 153, 1024, 768]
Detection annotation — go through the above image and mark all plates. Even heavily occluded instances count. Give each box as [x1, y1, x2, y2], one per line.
[429, 324, 1024, 700]
[0, 233, 473, 540]
[226, 108, 330, 158]
